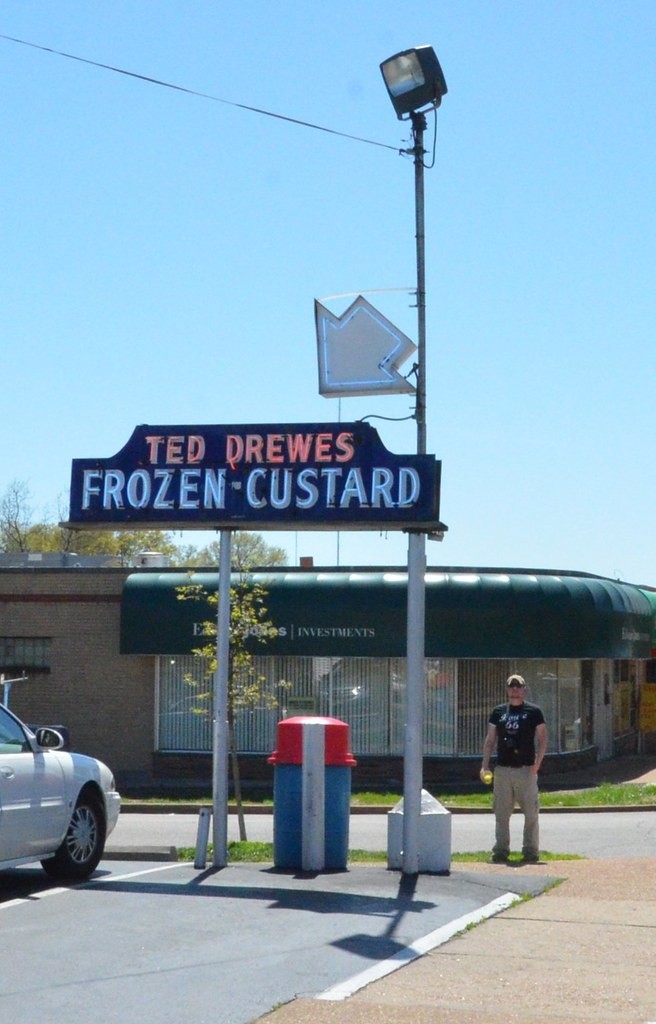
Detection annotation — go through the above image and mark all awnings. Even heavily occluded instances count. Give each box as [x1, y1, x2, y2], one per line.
[117, 567, 655, 662]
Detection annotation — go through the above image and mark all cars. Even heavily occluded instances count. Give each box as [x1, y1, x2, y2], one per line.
[0, 703, 122, 888]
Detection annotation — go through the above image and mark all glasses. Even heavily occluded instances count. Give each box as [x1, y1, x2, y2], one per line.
[508, 683, 522, 688]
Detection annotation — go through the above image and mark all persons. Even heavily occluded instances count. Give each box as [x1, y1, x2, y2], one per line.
[478, 674, 547, 864]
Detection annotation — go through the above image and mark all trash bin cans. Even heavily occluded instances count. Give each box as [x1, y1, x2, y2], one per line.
[267, 716, 357, 871]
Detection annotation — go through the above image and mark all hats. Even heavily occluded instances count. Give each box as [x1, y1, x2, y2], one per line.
[506, 674, 526, 686]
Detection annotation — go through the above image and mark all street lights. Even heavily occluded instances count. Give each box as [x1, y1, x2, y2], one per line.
[377, 44, 452, 879]
